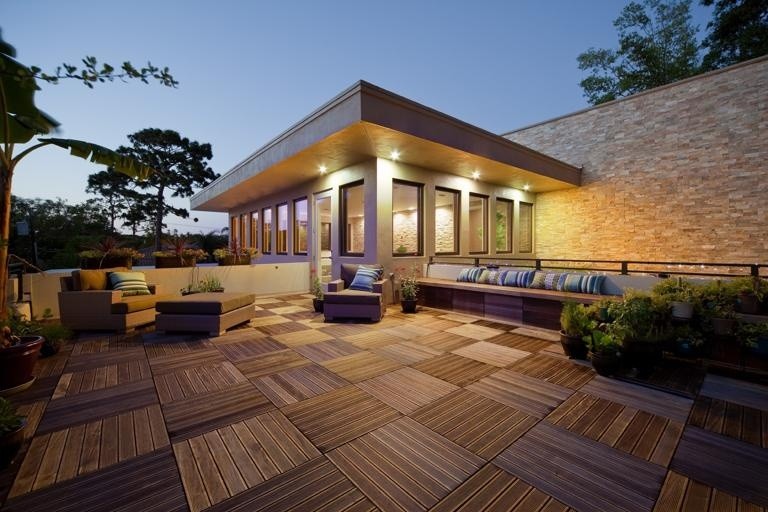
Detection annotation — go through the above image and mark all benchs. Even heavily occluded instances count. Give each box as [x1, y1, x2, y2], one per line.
[155, 292, 256, 337]
[402, 277, 624, 305]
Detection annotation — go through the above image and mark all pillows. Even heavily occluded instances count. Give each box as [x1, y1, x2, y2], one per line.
[72, 267, 153, 297]
[349, 264, 384, 293]
[456, 267, 606, 296]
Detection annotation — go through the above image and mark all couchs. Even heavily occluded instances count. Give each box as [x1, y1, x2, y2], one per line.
[57, 277, 177, 334]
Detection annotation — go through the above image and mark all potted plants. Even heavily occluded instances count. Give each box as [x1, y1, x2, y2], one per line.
[308, 277, 324, 312]
[78, 248, 145, 269]
[35, 320, 74, 356]
[152, 248, 209, 268]
[212, 248, 259, 266]
[0, 25, 165, 391]
[558, 274, 768, 377]
[0, 397, 28, 461]
[400, 277, 418, 313]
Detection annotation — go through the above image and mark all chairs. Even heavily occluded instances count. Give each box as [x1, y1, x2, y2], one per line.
[323, 263, 388, 322]
